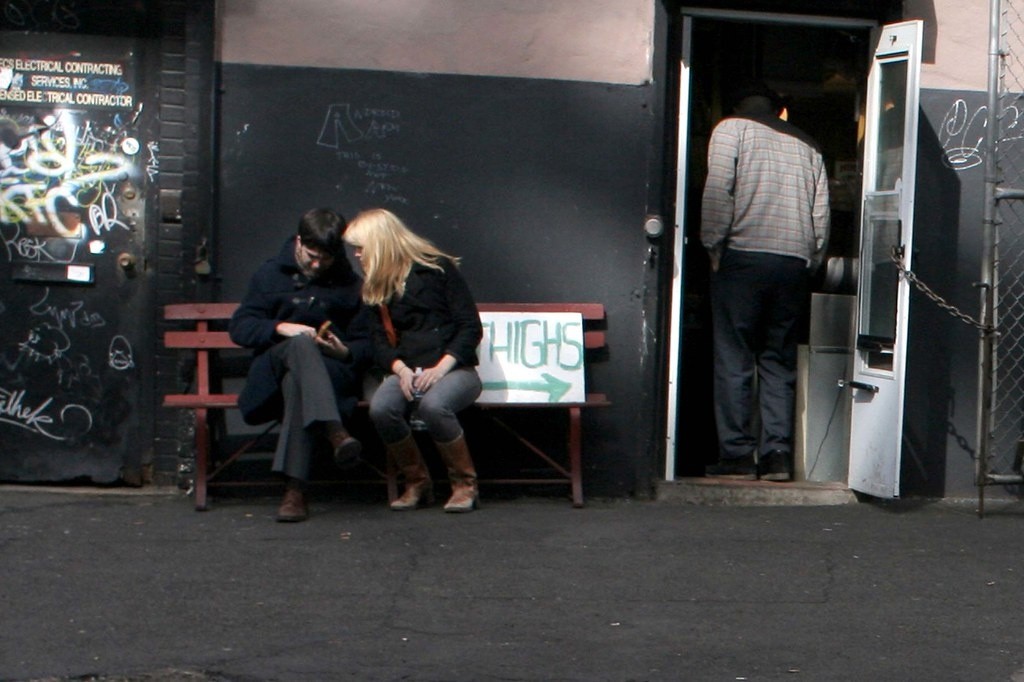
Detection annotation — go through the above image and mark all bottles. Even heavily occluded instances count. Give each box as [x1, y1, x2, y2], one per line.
[410, 366, 427, 430]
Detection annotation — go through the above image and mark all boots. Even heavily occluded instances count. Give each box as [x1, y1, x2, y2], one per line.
[388, 431, 433, 510]
[437, 428, 480, 515]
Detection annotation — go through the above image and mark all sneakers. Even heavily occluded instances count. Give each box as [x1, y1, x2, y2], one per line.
[704, 450, 790, 481]
[277, 483, 308, 524]
[329, 427, 362, 467]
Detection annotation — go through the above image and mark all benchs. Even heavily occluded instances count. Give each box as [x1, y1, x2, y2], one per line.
[163, 301, 609, 507]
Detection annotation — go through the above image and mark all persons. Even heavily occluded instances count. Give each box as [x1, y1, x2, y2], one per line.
[228, 207, 483, 523]
[698, 96, 831, 482]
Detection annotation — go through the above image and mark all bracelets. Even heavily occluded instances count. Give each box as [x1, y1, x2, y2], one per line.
[397, 365, 407, 375]
